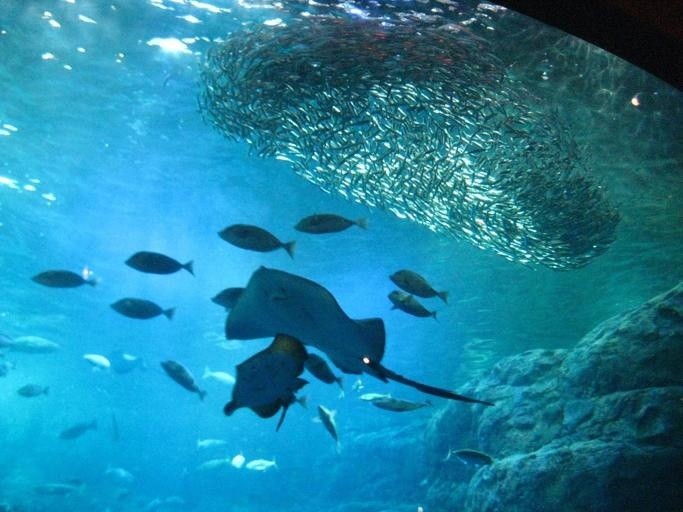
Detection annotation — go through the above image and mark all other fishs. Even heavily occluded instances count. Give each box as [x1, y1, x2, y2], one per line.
[191, 0, 626, 273]
[0, 332, 344, 511]
